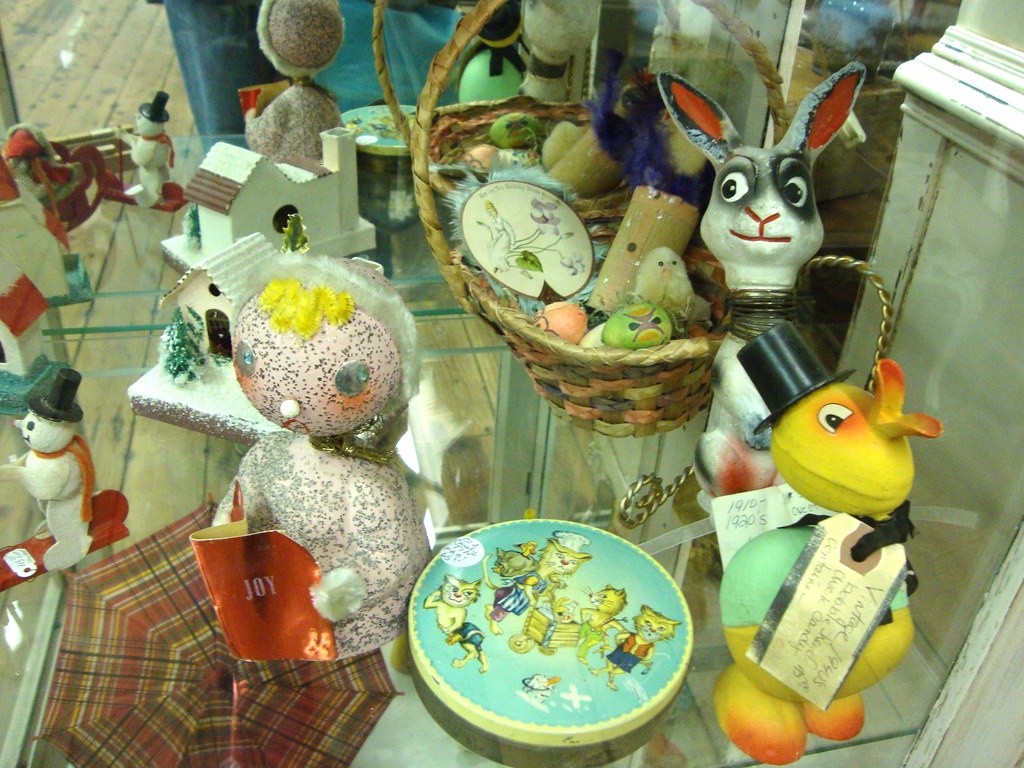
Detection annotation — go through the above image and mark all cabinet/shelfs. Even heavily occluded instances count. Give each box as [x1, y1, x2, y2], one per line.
[0, 136, 1023, 767]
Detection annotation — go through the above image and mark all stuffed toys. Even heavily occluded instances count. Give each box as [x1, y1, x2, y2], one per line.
[636, 245, 695, 318]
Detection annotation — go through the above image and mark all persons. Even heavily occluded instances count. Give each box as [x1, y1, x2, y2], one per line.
[204, 244, 441, 659]
[240, 0, 350, 179]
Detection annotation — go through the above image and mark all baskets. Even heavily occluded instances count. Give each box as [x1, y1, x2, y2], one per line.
[371, 0, 638, 209]
[412, 0, 793, 439]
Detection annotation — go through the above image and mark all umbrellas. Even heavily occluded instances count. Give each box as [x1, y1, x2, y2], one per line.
[27, 488, 405, 768]
